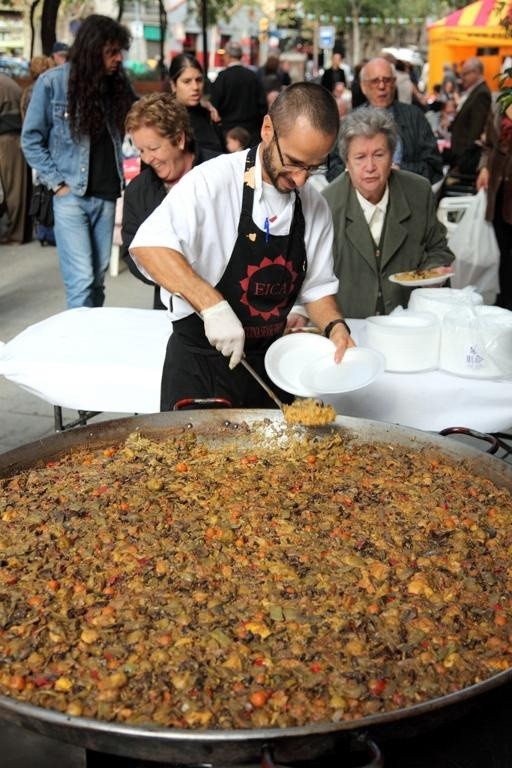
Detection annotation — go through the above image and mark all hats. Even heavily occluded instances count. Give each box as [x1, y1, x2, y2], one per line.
[52, 42, 70, 54]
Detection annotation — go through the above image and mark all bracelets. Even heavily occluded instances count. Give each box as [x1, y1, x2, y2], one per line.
[324, 318, 352, 338]
[50, 181, 65, 196]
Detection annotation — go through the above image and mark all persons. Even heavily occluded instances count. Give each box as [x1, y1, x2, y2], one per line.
[0, 73, 32, 245]
[140, 41, 491, 186]
[54, 39, 71, 68]
[18, 12, 223, 309]
[320, 103, 456, 320]
[475, 66, 511, 311]
[127, 80, 357, 412]
[120, 89, 225, 310]
[19, 53, 57, 248]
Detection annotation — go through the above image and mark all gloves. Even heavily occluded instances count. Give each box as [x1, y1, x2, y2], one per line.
[200, 300, 246, 370]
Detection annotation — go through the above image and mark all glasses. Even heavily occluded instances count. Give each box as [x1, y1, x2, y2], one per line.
[274, 129, 330, 174]
[361, 76, 396, 89]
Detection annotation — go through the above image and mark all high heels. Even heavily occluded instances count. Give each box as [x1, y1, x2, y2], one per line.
[39, 238, 56, 246]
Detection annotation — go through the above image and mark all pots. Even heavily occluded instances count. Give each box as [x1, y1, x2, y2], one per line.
[0, 396, 512, 768]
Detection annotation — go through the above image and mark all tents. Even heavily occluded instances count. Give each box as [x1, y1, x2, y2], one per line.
[427, 0, 512, 96]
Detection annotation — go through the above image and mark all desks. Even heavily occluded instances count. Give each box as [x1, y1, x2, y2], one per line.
[1, 307, 512, 432]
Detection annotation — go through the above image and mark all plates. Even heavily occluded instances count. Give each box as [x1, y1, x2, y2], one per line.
[263, 332, 337, 399]
[365, 315, 440, 373]
[307, 347, 384, 394]
[388, 270, 454, 287]
